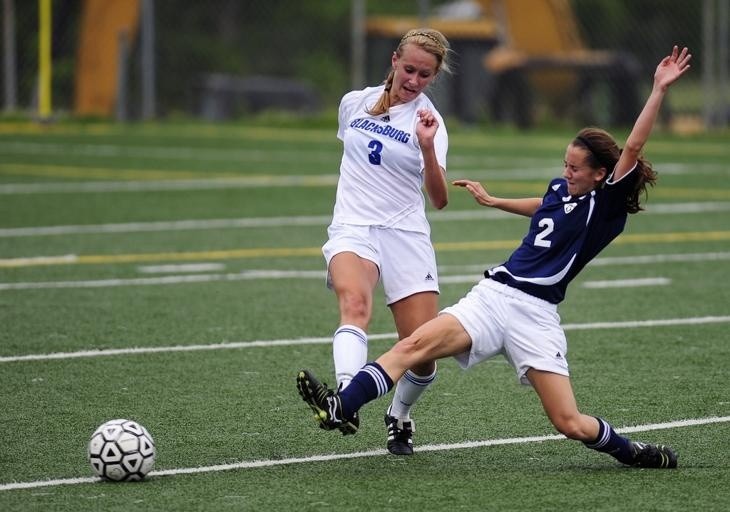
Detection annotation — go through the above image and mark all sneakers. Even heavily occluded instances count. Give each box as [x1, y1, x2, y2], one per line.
[384, 404, 416, 456]
[338, 412, 359, 436]
[631, 442, 677, 468]
[297, 370, 357, 431]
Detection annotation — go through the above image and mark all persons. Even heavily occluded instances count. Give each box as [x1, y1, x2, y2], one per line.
[502, 1, 641, 131]
[324, 26, 452, 456]
[435, 2, 535, 128]
[298, 47, 695, 466]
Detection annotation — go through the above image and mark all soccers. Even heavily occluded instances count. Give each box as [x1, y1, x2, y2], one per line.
[87, 419, 156, 483]
